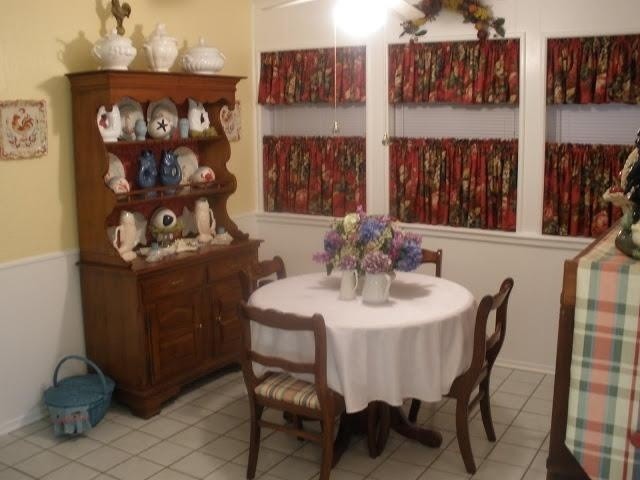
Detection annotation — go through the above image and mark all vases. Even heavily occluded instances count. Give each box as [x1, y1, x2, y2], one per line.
[340, 270, 358, 299]
[361, 272, 392, 304]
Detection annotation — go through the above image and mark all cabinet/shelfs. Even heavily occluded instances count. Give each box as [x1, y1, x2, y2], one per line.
[63, 68, 263, 421]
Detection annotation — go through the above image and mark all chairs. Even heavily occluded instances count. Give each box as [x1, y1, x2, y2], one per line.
[408, 277, 513, 474]
[237, 255, 286, 300]
[235, 296, 342, 480]
[420, 248, 442, 277]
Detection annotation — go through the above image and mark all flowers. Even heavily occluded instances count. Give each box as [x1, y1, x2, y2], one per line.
[313, 205, 423, 276]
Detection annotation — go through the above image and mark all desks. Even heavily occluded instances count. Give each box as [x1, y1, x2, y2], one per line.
[545, 214, 639, 479]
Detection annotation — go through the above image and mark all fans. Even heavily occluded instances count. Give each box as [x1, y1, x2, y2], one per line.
[264, 0, 426, 22]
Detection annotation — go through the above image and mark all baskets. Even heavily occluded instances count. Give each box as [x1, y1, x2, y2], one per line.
[43, 356, 115, 434]
